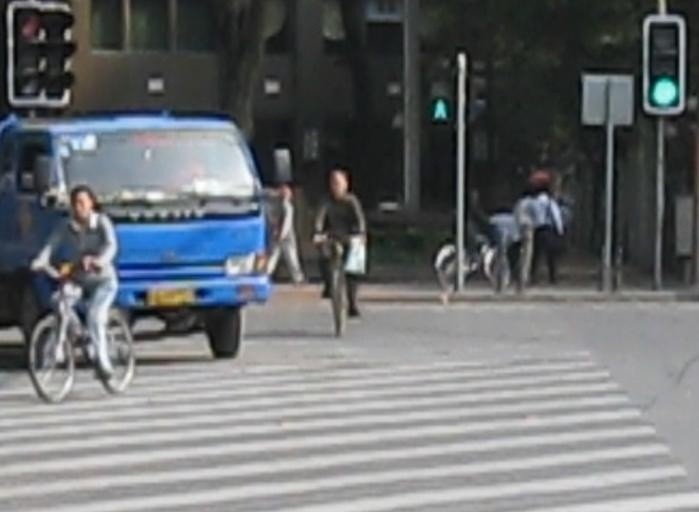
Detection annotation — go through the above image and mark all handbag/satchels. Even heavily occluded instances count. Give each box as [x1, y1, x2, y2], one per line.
[343, 236, 368, 276]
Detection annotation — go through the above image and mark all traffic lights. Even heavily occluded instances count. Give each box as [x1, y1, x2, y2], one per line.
[8, 2, 77, 107]
[642, 16, 686, 115]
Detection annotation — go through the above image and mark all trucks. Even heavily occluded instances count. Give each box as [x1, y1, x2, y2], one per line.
[0, 112, 270, 367]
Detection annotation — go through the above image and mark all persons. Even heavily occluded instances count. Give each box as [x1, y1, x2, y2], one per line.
[528, 183, 564, 282]
[26, 181, 121, 381]
[514, 191, 532, 285]
[489, 206, 523, 294]
[458, 189, 492, 246]
[314, 167, 366, 323]
[260, 185, 308, 287]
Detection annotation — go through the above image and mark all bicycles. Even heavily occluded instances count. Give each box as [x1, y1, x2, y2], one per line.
[434, 234, 497, 291]
[28, 262, 136, 404]
[315, 230, 366, 336]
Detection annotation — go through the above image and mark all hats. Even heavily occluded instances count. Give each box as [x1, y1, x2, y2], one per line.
[532, 169, 551, 186]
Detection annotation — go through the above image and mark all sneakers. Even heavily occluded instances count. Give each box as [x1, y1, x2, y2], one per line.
[43, 343, 114, 380]
[293, 272, 360, 317]
[528, 276, 561, 286]
